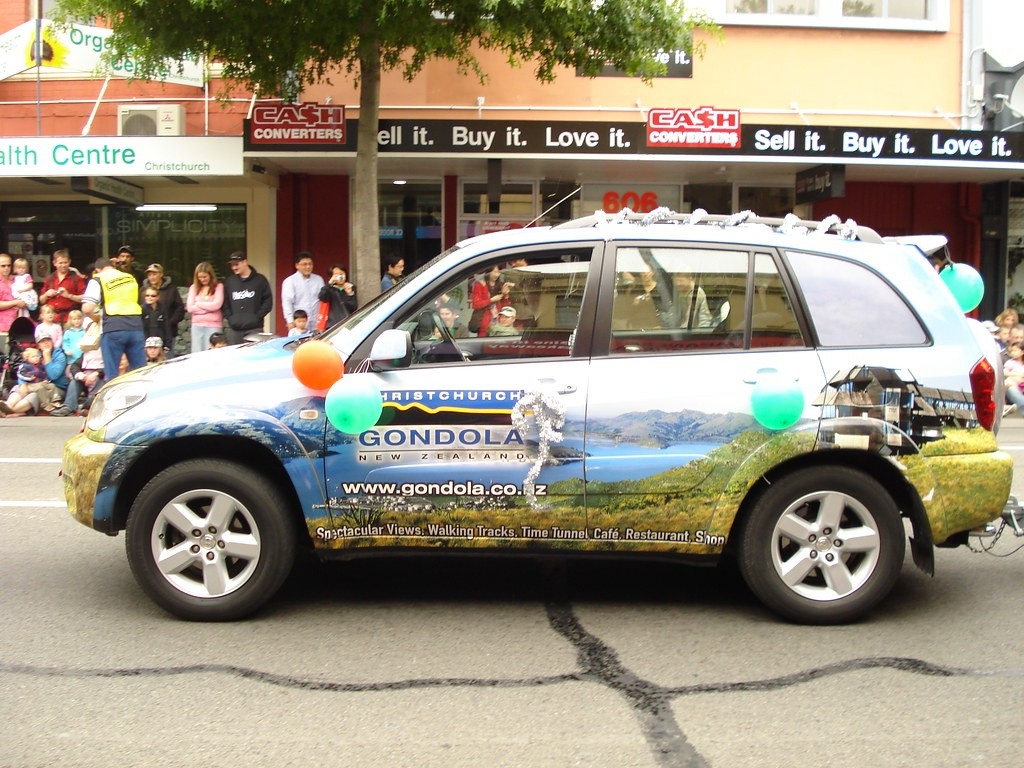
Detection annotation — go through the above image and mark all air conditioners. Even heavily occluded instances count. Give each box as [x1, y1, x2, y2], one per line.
[117, 103, 187, 137]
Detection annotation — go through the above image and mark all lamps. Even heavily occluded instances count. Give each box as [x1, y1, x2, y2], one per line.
[130, 204, 218, 212]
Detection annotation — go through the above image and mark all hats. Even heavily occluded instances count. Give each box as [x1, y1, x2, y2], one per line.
[118, 245, 135, 256]
[498, 306, 516, 317]
[143, 336, 163, 348]
[144, 263, 163, 273]
[35, 331, 52, 344]
[981, 320, 1000, 332]
[226, 251, 245, 265]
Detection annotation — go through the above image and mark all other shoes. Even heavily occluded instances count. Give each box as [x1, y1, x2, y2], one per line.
[42, 401, 62, 411]
[1002, 403, 1017, 418]
[80, 409, 88, 415]
[50, 405, 74, 417]
[0, 400, 16, 418]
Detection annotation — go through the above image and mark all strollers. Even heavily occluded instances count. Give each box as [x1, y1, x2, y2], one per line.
[0, 317, 38, 399]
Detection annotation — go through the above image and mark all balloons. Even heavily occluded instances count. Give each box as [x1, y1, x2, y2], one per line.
[751, 372, 805, 430]
[324, 373, 383, 434]
[939, 263, 984, 313]
[292, 341, 343, 391]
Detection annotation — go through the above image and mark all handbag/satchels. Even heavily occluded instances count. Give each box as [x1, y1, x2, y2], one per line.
[468, 308, 485, 332]
[79, 305, 104, 352]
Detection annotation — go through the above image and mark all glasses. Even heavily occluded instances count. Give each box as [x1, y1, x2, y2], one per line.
[145, 295, 158, 297]
[230, 256, 244, 260]
[0, 264, 12, 267]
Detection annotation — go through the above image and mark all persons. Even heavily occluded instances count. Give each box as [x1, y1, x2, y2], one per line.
[617, 262, 713, 329]
[926, 256, 941, 274]
[428, 257, 566, 340]
[281, 252, 358, 338]
[382, 254, 404, 294]
[981, 309, 1024, 418]
[0, 245, 272, 415]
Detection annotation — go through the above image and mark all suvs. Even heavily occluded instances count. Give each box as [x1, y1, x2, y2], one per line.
[60, 206, 1024, 627]
